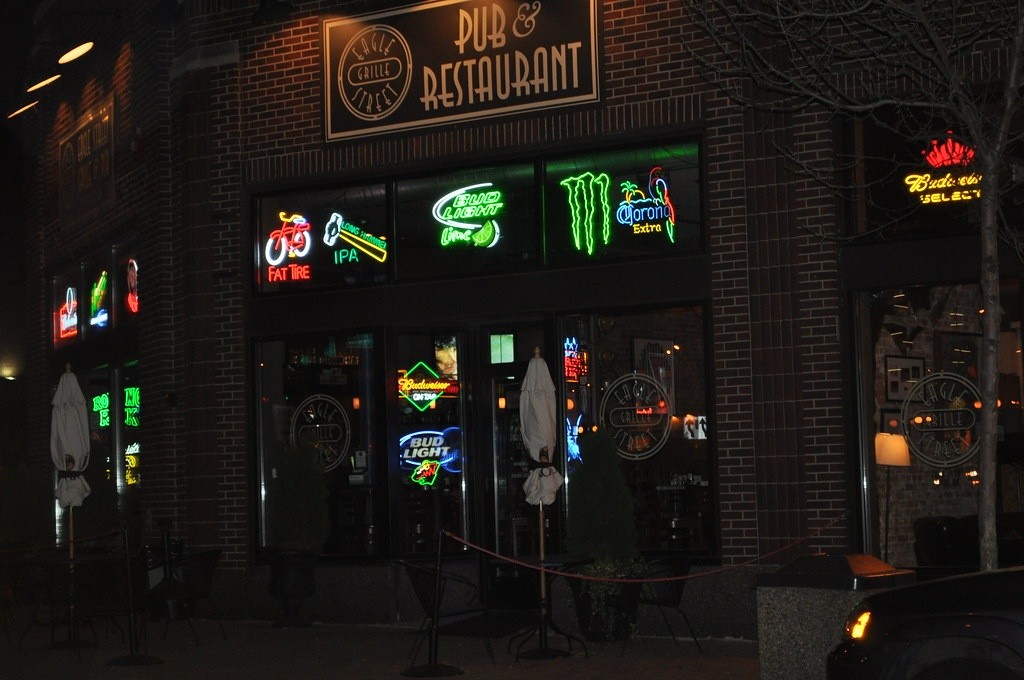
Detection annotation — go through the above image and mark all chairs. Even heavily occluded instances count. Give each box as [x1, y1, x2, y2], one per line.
[596, 555, 705, 657]
[1, 540, 229, 664]
[398, 559, 496, 669]
[623, 482, 704, 555]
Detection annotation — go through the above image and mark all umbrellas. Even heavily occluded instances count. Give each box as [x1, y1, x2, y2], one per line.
[50, 362, 92, 568]
[520, 348, 565, 614]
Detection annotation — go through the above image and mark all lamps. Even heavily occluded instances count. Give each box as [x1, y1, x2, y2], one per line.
[874, 431, 912, 565]
[0, 7, 122, 119]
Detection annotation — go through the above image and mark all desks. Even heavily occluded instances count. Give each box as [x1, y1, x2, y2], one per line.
[489, 555, 599, 663]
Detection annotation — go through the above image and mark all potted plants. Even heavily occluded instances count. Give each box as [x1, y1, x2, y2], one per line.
[565, 558, 658, 650]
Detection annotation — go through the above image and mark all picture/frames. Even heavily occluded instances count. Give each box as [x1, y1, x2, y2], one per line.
[629, 335, 679, 418]
[884, 355, 926, 403]
[881, 408, 905, 433]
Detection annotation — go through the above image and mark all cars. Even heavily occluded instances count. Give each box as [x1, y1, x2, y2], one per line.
[826, 566, 1024, 680]
